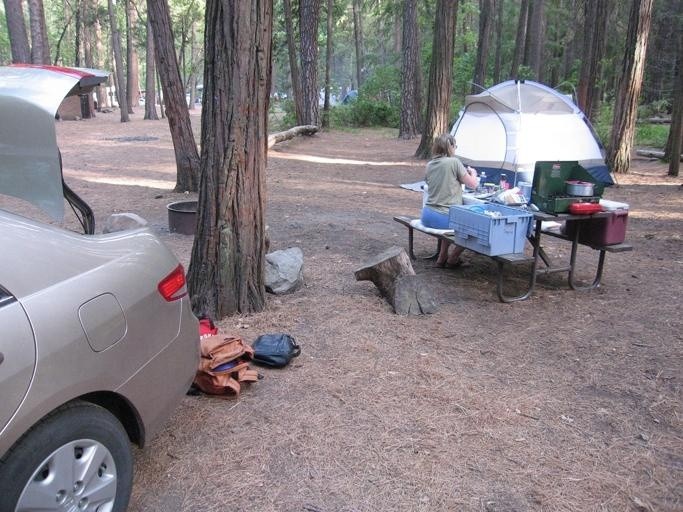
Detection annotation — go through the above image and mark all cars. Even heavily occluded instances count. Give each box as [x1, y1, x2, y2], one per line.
[1, 61, 207, 511]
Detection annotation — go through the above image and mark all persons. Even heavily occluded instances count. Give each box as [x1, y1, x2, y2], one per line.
[417, 135, 479, 271]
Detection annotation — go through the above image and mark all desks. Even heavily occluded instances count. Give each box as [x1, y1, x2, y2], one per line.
[462, 185, 612, 304]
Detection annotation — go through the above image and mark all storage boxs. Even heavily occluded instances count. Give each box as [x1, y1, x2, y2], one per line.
[565, 198, 628, 246]
[447, 203, 534, 258]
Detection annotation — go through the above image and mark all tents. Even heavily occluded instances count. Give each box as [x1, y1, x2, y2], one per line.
[448, 76, 615, 202]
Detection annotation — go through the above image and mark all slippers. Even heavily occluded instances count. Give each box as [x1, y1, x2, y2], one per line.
[432, 257, 464, 271]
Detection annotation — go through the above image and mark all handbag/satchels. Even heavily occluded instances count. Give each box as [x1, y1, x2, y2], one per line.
[251, 332, 301, 368]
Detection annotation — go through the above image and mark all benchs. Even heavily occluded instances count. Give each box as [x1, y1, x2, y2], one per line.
[532, 221, 631, 291]
[392, 215, 536, 304]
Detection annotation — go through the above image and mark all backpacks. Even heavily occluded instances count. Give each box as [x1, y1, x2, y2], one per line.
[189, 313, 259, 406]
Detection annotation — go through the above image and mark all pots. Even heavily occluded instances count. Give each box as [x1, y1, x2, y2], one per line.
[564, 179, 598, 197]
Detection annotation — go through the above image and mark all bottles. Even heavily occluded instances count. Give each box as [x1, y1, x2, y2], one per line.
[476, 172, 532, 201]
[421, 186, 429, 190]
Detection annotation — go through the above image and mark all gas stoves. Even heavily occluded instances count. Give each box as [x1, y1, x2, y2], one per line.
[530, 192, 600, 213]
